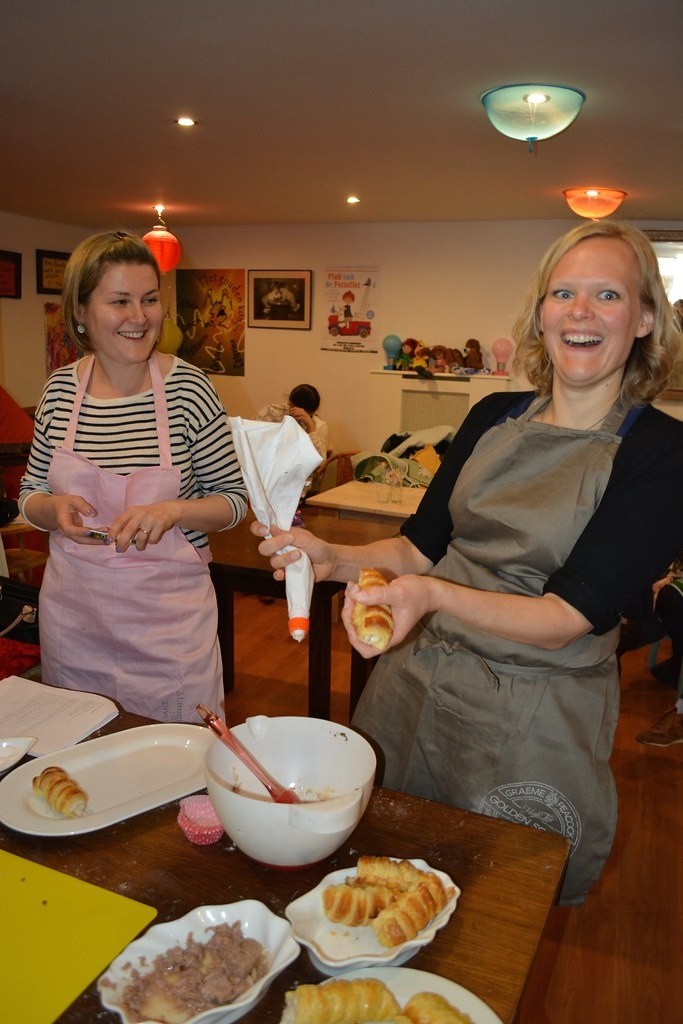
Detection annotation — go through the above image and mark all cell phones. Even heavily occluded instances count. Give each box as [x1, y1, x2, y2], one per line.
[90, 529, 136, 545]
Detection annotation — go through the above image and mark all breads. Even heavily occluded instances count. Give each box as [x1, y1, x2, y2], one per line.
[352, 567, 393, 651]
[31, 766, 88, 818]
[281, 978, 474, 1024]
[322, 856, 448, 947]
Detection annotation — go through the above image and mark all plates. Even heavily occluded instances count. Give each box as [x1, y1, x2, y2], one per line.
[0, 736, 38, 778]
[285, 855, 462, 969]
[0, 723, 223, 837]
[279, 966, 506, 1023]
[97, 899, 301, 1023]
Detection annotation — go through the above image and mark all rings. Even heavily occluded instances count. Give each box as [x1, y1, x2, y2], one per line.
[138, 527, 149, 534]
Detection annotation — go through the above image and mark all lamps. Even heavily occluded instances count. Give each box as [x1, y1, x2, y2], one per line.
[562, 187, 628, 221]
[141, 203, 183, 276]
[479, 82, 587, 154]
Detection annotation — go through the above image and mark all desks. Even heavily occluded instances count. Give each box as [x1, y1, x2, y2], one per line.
[207, 508, 401, 724]
[304, 481, 427, 624]
[0, 708, 571, 1024]
[368, 369, 512, 452]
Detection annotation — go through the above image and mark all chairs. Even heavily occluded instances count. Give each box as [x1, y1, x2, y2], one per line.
[316, 450, 363, 494]
[0, 505, 51, 586]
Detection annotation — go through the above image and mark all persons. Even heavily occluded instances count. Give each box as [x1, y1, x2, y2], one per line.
[616, 562, 683, 747]
[249, 219, 683, 909]
[17, 228, 250, 731]
[253, 384, 328, 500]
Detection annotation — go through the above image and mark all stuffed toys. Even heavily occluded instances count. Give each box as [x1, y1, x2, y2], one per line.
[396, 336, 484, 377]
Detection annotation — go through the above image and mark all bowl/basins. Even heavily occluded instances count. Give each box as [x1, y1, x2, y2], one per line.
[205, 715, 378, 868]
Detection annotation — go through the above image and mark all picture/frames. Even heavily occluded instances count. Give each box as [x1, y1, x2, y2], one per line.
[35, 248, 71, 295]
[246, 269, 313, 331]
[0, 249, 22, 299]
